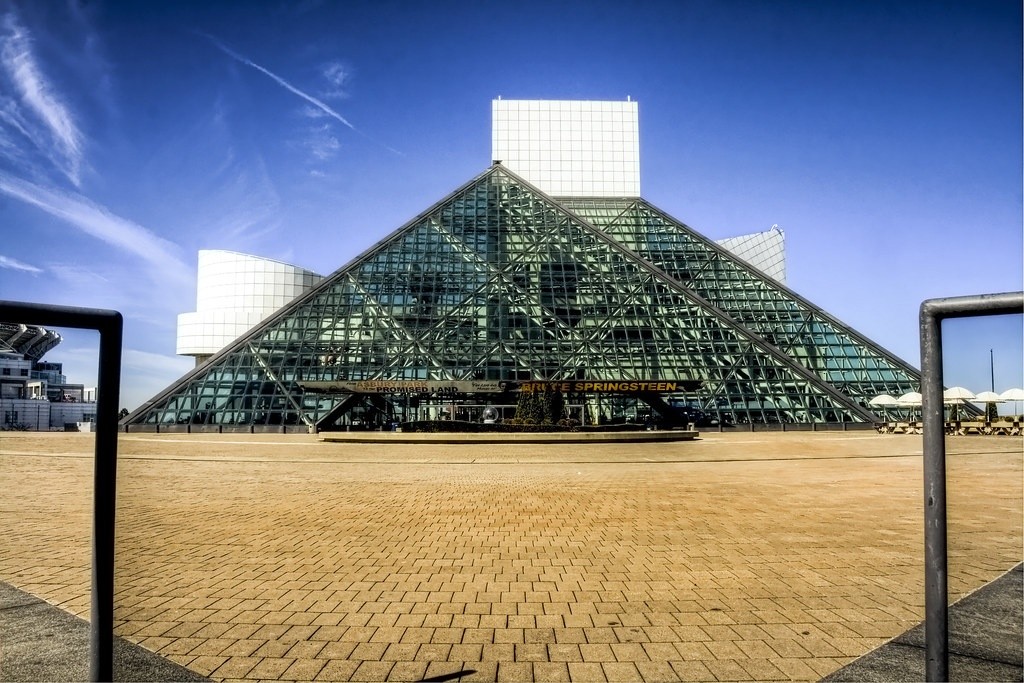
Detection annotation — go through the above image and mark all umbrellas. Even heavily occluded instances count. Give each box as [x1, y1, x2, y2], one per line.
[896, 386, 977, 423]
[868, 395, 899, 423]
[969, 391, 1007, 422]
[996, 388, 1024, 416]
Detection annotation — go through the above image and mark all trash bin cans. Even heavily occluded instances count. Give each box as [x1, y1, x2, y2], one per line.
[688, 422, 695, 430]
[309, 423, 315, 433]
[392, 422, 399, 431]
